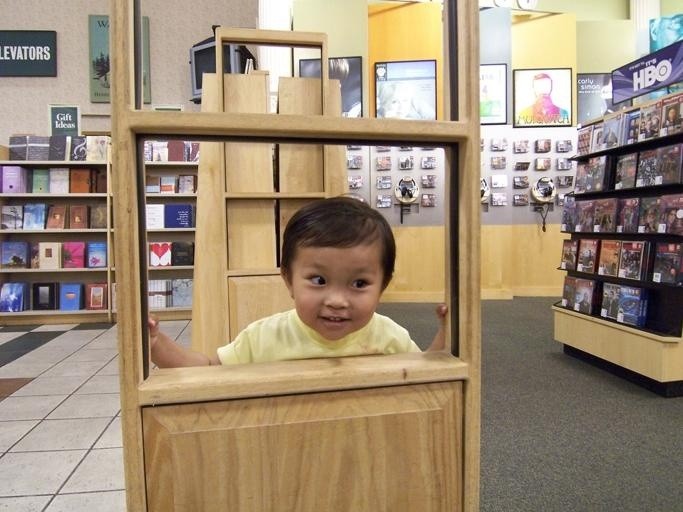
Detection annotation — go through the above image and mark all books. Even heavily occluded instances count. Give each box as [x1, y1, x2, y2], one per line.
[144, 141, 199, 307]
[480, 139, 573, 206]
[348, 145, 436, 207]
[560, 93, 683, 329]
[1, 136, 117, 312]
[50, 104, 80, 136]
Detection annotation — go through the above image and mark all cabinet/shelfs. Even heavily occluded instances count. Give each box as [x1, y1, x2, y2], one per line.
[0, 157, 111, 323]
[188, 25, 349, 366]
[547, 88, 683, 399]
[108, 160, 201, 321]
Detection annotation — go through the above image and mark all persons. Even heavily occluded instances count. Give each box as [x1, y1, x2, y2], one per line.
[147, 196, 448, 369]
[518, 73, 568, 124]
[376, 79, 435, 118]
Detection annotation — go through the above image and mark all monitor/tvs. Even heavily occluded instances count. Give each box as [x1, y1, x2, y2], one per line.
[189, 38, 257, 105]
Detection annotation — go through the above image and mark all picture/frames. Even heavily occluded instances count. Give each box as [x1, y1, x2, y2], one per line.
[513, 68, 571, 130]
[575, 72, 631, 130]
[296, 56, 363, 120]
[478, 62, 509, 126]
[86, 13, 152, 104]
[0, 29, 57, 78]
[374, 58, 438, 123]
